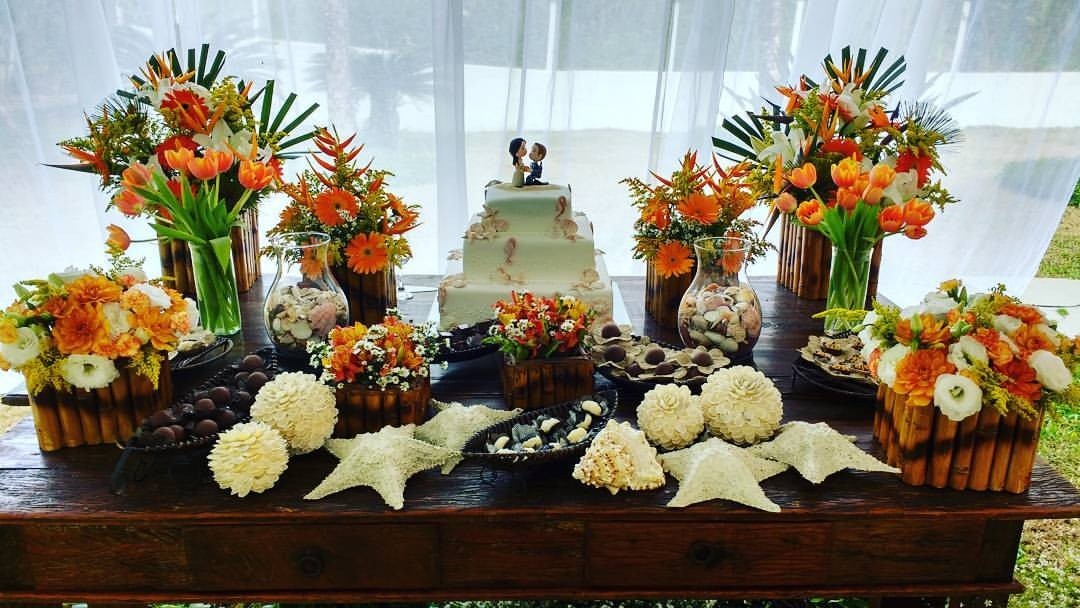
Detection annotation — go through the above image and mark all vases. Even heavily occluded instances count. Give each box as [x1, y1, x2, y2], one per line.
[677, 243, 766, 359]
[155, 204, 264, 299]
[499, 360, 602, 413]
[641, 239, 740, 331]
[870, 378, 1047, 498]
[264, 228, 352, 362]
[821, 243, 878, 338]
[296, 258, 402, 318]
[22, 361, 172, 452]
[324, 371, 439, 429]
[775, 212, 884, 307]
[181, 238, 246, 341]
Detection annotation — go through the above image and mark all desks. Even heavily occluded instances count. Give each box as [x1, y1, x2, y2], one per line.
[0, 281, 1080, 608]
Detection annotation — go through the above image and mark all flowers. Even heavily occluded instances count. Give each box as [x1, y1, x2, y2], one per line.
[615, 46, 1080, 434]
[0, 41, 450, 396]
[479, 284, 600, 354]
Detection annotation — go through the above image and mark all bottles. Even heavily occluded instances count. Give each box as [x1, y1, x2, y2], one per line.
[677, 237, 762, 359]
[263, 232, 349, 357]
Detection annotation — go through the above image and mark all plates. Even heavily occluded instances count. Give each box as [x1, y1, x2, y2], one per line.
[461, 390, 619, 465]
[792, 356, 876, 397]
[429, 318, 501, 364]
[582, 333, 707, 386]
[169, 337, 234, 370]
[116, 345, 278, 451]
